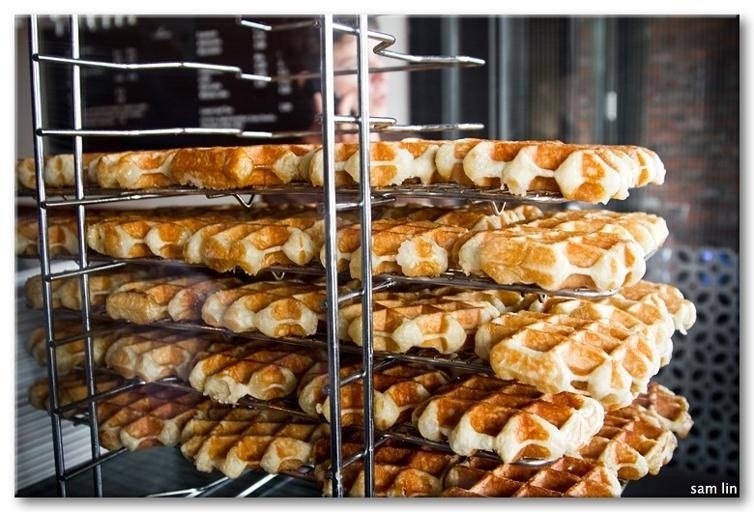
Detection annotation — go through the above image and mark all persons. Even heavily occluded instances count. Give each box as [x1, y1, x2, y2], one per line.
[267, 14, 390, 148]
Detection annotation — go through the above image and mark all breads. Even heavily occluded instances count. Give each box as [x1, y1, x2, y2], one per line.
[17, 138, 697, 497]
